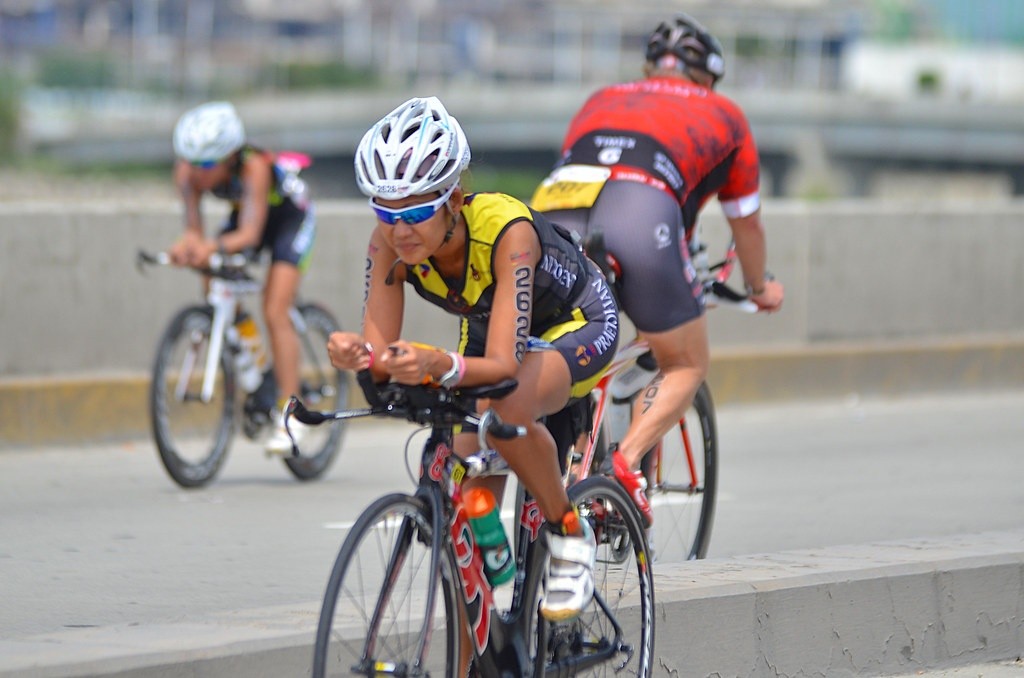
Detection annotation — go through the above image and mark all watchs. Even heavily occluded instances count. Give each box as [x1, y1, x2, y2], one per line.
[435, 347, 456, 386]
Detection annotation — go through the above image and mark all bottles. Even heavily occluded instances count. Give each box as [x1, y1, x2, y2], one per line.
[235, 312, 272, 375]
[463, 486, 520, 589]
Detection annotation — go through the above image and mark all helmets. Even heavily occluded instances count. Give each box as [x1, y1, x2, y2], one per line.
[353, 94, 472, 200]
[646, 14, 724, 82]
[172, 101, 248, 161]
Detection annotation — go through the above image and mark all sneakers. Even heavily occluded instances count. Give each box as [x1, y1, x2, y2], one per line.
[264, 412, 305, 454]
[540, 519, 599, 620]
[599, 453, 653, 528]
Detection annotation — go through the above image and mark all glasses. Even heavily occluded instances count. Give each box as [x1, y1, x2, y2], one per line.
[186, 160, 216, 170]
[367, 176, 460, 226]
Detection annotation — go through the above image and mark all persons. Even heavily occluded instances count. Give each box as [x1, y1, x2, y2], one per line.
[175, 101, 316, 459]
[529, 13, 783, 530]
[326, 94, 622, 678]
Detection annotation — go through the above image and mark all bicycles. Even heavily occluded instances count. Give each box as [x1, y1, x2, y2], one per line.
[282, 366, 659, 678]
[565, 221, 758, 567]
[134, 240, 356, 486]
